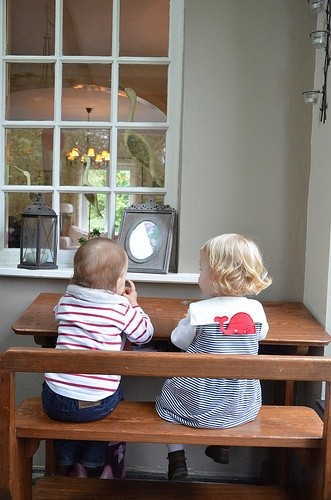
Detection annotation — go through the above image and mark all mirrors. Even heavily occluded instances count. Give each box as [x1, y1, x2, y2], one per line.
[116, 199, 176, 274]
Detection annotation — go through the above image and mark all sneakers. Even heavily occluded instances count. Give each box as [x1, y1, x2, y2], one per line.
[205, 445, 229, 464]
[167, 450, 188, 483]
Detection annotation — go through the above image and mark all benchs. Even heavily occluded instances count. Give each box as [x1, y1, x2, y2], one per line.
[0, 347, 331, 500]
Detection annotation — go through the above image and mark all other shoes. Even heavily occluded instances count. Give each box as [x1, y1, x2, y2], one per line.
[79, 458, 104, 468]
[56, 455, 77, 466]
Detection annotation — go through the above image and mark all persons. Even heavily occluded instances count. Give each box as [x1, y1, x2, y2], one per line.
[155, 234, 272, 481]
[41, 237, 154, 480]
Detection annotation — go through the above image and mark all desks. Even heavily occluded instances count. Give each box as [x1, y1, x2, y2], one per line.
[12, 292, 331, 483]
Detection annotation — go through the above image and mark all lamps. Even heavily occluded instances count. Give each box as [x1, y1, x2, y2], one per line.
[302, 0, 331, 124]
[16, 192, 58, 269]
[66, 108, 111, 163]
